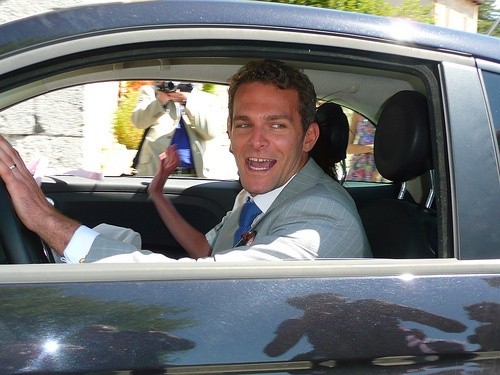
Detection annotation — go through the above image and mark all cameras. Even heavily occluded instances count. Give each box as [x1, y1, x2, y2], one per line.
[155, 80, 193, 93]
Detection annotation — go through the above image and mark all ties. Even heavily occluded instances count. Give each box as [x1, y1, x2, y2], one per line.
[234, 202, 261, 244]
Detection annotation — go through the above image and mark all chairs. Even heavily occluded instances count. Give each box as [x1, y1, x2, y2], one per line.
[355, 90, 440, 258]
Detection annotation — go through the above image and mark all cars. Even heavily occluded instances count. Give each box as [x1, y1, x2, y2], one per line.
[0, 0, 500, 375]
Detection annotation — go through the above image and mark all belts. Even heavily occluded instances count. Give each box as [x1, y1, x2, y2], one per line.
[172, 169, 191, 173]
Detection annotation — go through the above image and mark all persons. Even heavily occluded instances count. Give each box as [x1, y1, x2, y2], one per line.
[0, 59, 373, 264]
[131, 79, 214, 178]
[345, 111, 395, 183]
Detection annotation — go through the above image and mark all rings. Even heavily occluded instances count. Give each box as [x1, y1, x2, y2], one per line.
[9, 164, 17, 170]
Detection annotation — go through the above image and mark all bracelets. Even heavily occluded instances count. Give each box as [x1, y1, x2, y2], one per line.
[182, 97, 187, 106]
